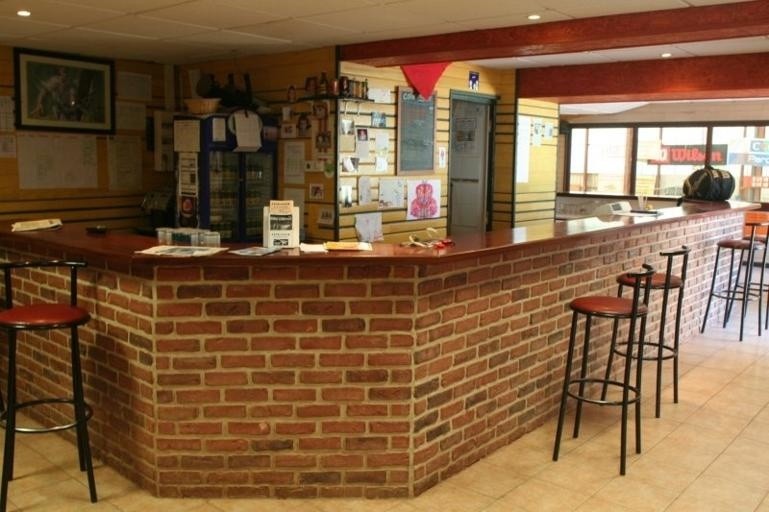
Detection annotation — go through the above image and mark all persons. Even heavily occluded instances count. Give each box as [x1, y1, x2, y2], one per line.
[306, 78, 315, 96]
[284, 126, 294, 133]
[471, 71, 480, 91]
[313, 102, 330, 119]
[316, 134, 326, 148]
[311, 185, 322, 197]
[359, 130, 367, 141]
[326, 131, 331, 147]
[264, 131, 273, 138]
[288, 87, 297, 101]
[30, 64, 100, 122]
[297, 115, 312, 137]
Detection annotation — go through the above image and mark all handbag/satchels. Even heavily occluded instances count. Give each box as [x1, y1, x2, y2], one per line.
[676, 167, 735, 208]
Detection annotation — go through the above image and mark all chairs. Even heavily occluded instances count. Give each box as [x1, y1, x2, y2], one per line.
[0, 259, 100, 512]
[699, 220, 768, 341]
[599, 244, 693, 419]
[551, 264, 655, 476]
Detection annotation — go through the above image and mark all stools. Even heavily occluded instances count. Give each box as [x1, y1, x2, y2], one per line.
[727, 234, 769, 336]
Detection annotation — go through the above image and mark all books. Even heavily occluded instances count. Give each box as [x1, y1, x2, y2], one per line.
[157, 227, 221, 247]
[268, 212, 295, 249]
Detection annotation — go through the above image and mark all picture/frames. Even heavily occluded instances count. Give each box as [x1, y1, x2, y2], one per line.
[12, 46, 116, 135]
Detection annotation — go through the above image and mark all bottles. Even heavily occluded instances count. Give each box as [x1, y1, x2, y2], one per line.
[319, 71, 328, 96]
